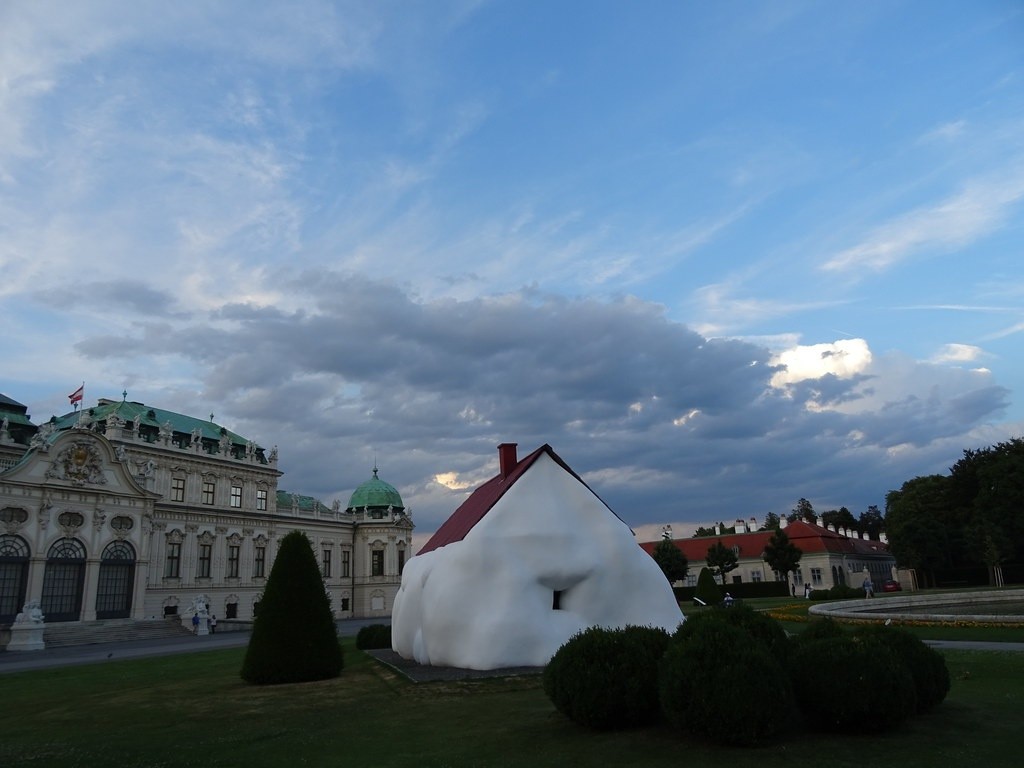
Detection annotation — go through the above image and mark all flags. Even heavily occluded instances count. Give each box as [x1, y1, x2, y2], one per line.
[68, 385, 84, 405]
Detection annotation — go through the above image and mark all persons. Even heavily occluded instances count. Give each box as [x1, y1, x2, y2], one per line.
[792, 583, 797, 598]
[2, 415, 9, 430]
[210, 615, 217, 634]
[192, 613, 201, 635]
[863, 577, 873, 599]
[804, 583, 814, 598]
[94, 511, 106, 530]
[145, 460, 158, 477]
[725, 592, 733, 606]
[158, 420, 279, 460]
[291, 492, 413, 518]
[133, 414, 141, 430]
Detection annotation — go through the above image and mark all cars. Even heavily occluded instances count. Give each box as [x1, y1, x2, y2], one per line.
[883, 582, 901, 591]
